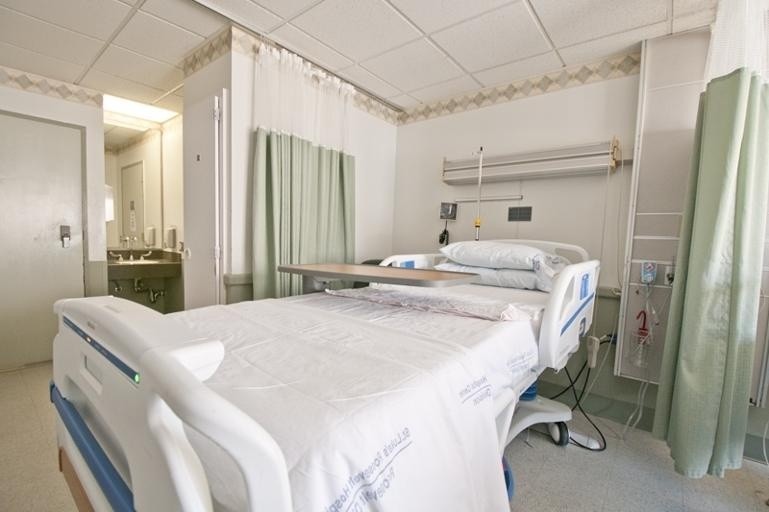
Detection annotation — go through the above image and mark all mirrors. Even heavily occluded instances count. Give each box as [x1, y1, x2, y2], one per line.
[103, 122, 166, 251]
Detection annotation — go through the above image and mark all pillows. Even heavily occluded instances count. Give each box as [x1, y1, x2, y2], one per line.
[439, 241, 550, 270]
[434, 259, 564, 290]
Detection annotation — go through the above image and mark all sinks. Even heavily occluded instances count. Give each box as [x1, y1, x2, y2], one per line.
[116, 259, 157, 264]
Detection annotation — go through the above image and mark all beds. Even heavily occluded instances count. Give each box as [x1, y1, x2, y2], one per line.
[45, 240, 602, 512]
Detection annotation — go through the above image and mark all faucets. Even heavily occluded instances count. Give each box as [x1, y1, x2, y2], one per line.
[128, 236, 138, 260]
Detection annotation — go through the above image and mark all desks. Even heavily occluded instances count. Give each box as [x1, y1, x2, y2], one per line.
[273, 262, 481, 297]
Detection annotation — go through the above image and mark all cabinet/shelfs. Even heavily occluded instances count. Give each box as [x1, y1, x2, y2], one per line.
[606, 210, 683, 389]
